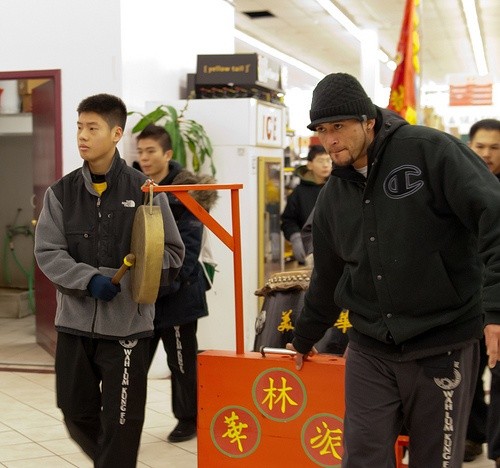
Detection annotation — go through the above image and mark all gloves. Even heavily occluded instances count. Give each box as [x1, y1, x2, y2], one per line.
[86, 274, 120, 302]
[290, 233, 307, 265]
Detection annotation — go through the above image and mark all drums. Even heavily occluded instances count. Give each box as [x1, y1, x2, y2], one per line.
[253, 269, 349, 355]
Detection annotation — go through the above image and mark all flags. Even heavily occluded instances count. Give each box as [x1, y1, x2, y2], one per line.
[389, 0, 420, 126]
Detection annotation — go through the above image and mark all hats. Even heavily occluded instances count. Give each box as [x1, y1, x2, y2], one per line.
[306, 73, 378, 131]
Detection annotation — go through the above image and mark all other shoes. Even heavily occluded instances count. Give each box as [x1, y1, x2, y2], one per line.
[462, 440, 483, 462]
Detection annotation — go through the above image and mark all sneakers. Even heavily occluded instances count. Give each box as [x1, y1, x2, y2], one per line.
[166, 420, 196, 442]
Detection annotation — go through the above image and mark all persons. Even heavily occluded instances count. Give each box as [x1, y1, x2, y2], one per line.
[287, 73, 500, 468]
[280, 146, 334, 262]
[465, 119, 500, 461]
[32, 95, 185, 468]
[130, 126, 219, 443]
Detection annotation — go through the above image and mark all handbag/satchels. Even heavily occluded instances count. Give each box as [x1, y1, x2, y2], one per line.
[198, 260, 217, 289]
